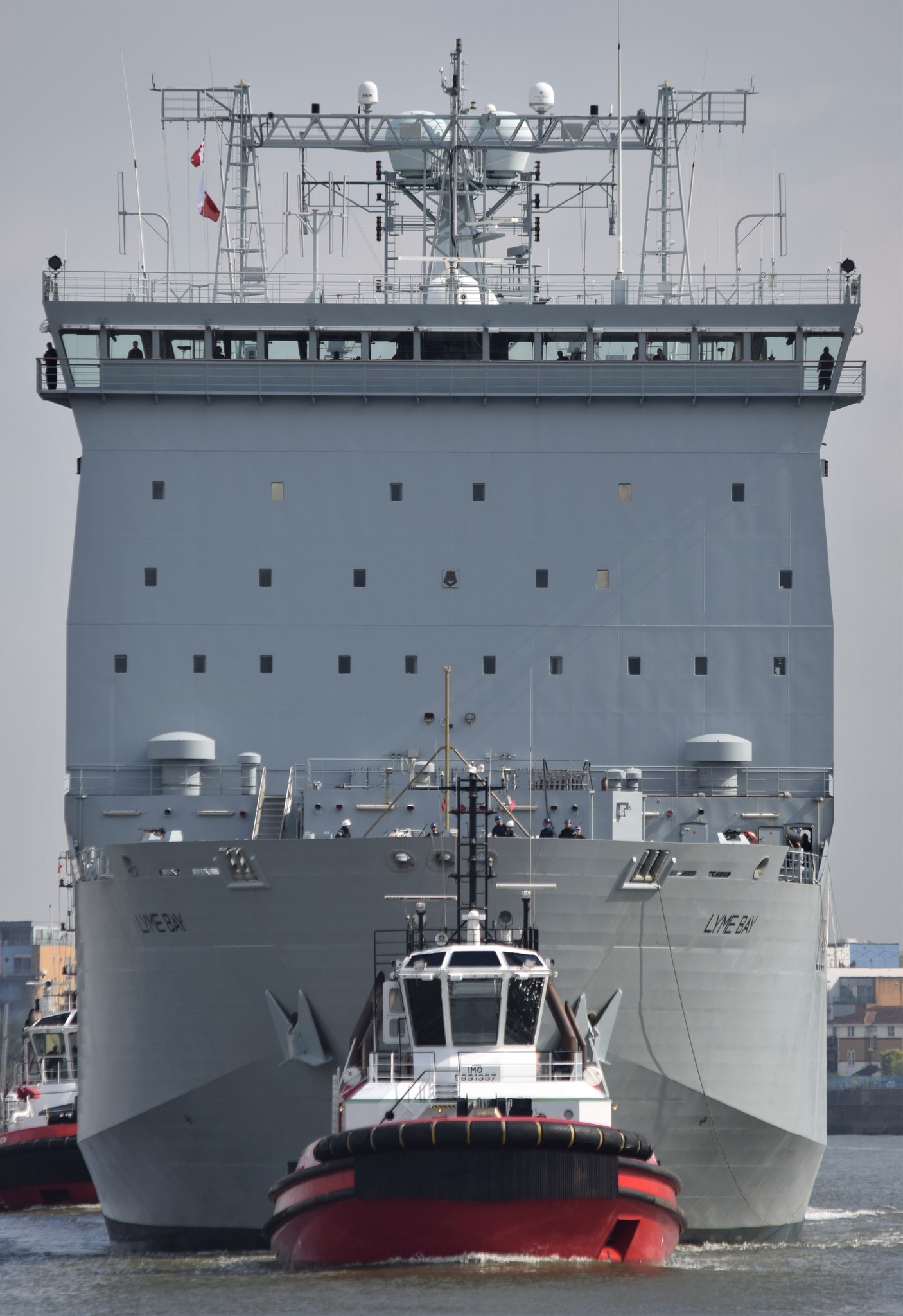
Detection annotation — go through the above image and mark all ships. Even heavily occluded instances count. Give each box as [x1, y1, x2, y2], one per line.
[36, 27, 867, 1253]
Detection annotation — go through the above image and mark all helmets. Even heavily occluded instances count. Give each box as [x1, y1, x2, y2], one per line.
[564, 819, 571, 824]
[494, 815, 502, 821]
[543, 818, 551, 824]
[506, 821, 514, 827]
[430, 823, 437, 827]
[341, 820, 351, 826]
[575, 826, 581, 830]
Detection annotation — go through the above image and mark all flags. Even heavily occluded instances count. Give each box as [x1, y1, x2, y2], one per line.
[57, 864, 62, 874]
[190, 136, 205, 168]
[508, 795, 515, 813]
[440, 797, 446, 812]
[197, 173, 221, 223]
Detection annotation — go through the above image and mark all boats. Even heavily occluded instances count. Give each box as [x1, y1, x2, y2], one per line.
[0, 954, 99, 1210]
[263, 661, 689, 1270]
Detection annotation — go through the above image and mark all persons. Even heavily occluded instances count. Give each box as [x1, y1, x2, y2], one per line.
[335, 819, 352, 838]
[492, 815, 519, 838]
[539, 818, 555, 838]
[393, 348, 404, 360]
[558, 819, 587, 840]
[330, 351, 343, 360]
[570, 347, 584, 361]
[127, 341, 143, 358]
[632, 347, 638, 361]
[817, 347, 834, 390]
[212, 346, 225, 359]
[556, 350, 570, 361]
[652, 348, 667, 361]
[426, 823, 439, 837]
[790, 834, 811, 867]
[43, 342, 58, 390]
[45, 1046, 63, 1079]
